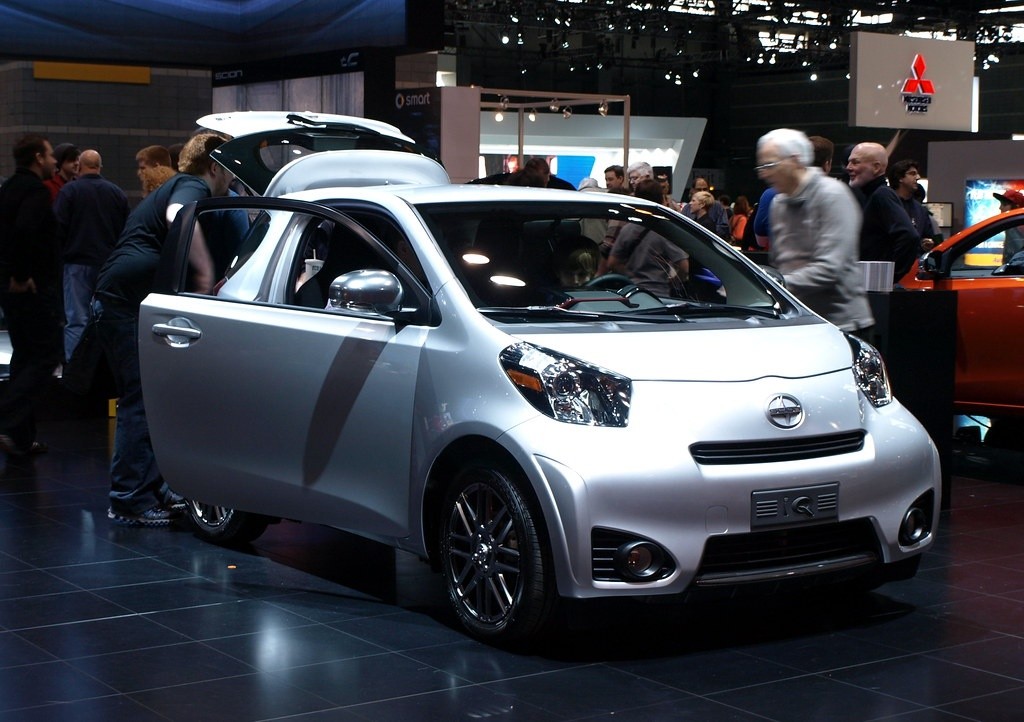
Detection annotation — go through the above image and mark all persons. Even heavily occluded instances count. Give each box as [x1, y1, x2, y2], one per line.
[605, 164, 626, 191]
[886, 162, 935, 245]
[682, 176, 730, 241]
[689, 192, 716, 232]
[607, 180, 690, 297]
[729, 196, 749, 240]
[915, 184, 943, 247]
[1, 132, 252, 525]
[572, 177, 607, 244]
[627, 162, 656, 196]
[521, 158, 551, 188]
[756, 128, 877, 341]
[993, 190, 1024, 265]
[846, 142, 920, 280]
[753, 137, 831, 251]
[718, 193, 733, 216]
[546, 247, 596, 306]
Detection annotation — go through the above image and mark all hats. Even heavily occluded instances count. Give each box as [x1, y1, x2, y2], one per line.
[53, 142, 77, 169]
[577, 177, 601, 190]
[993, 189, 1024, 208]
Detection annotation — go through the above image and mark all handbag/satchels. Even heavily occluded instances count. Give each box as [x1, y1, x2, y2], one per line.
[715, 201, 730, 240]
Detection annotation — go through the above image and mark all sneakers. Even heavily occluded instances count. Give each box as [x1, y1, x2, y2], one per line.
[159, 488, 188, 509]
[107, 507, 171, 528]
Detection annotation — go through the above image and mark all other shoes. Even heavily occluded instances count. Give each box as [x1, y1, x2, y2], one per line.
[0, 435, 24, 455]
[27, 442, 40, 452]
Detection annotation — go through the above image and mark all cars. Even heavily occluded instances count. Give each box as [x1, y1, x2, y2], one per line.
[136, 106, 944, 639]
[894, 206, 1024, 412]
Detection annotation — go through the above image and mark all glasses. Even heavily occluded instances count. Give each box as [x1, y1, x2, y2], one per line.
[628, 176, 642, 182]
[695, 187, 708, 191]
[755, 159, 783, 173]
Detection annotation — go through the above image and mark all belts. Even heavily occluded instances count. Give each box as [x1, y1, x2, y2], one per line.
[93, 291, 129, 307]
